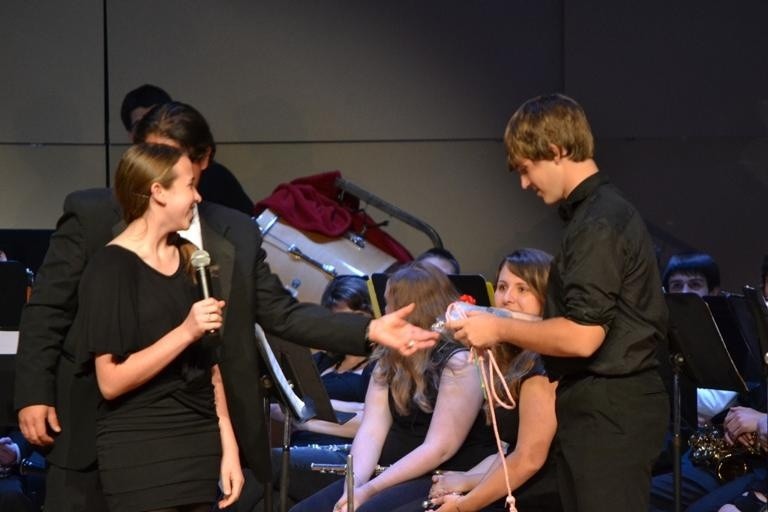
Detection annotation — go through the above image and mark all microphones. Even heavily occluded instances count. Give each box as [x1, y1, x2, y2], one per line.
[190, 250, 221, 336]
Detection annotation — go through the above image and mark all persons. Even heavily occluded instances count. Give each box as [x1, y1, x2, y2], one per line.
[649, 249, 768, 512]
[65, 140, 246, 512]
[270, 248, 572, 512]
[444, 94, 672, 512]
[120, 85, 254, 215]
[13, 102, 439, 512]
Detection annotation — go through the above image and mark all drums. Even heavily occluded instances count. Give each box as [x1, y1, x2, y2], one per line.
[255, 207, 398, 304]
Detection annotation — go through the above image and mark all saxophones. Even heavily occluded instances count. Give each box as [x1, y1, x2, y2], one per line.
[687, 431, 752, 484]
